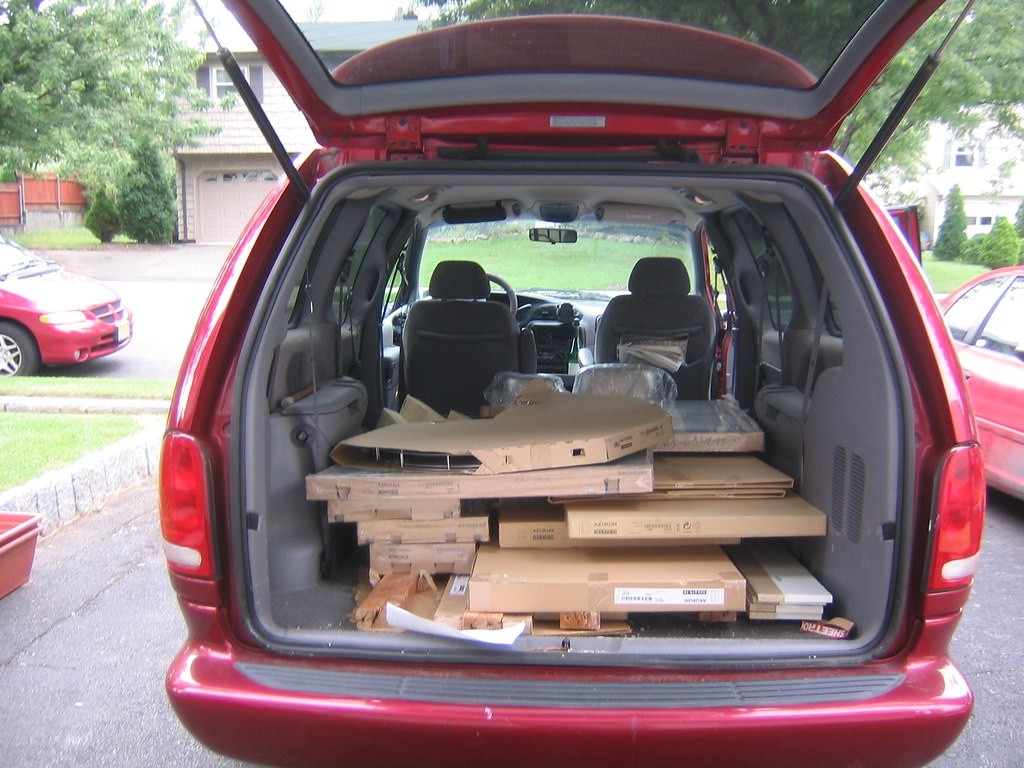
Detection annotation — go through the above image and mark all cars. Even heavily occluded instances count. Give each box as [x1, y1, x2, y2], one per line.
[936, 268, 1024, 502]
[0, 235, 133, 375]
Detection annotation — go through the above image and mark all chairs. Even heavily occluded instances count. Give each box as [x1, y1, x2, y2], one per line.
[593, 258, 715, 401]
[401, 261, 538, 418]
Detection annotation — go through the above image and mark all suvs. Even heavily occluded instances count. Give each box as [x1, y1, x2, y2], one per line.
[158, 0, 987, 768]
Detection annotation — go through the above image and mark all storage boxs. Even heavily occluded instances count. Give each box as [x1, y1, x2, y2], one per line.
[304, 395, 828, 630]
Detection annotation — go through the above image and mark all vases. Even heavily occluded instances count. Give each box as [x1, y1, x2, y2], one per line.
[0, 511, 43, 598]
